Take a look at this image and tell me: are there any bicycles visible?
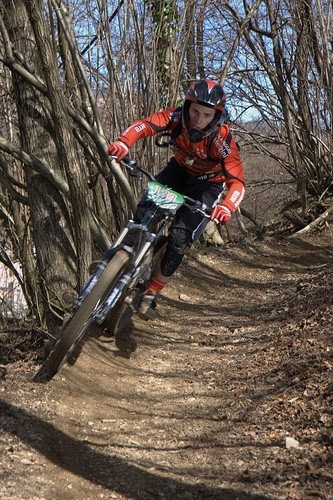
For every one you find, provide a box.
[46,152,220,377]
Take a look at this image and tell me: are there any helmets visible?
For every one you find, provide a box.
[182,80,225,143]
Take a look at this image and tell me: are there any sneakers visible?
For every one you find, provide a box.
[137,290,158,321]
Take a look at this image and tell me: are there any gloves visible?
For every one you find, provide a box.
[108,141,129,164]
[210,205,231,225]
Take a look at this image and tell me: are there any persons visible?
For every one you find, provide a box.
[88,78,246,316]
